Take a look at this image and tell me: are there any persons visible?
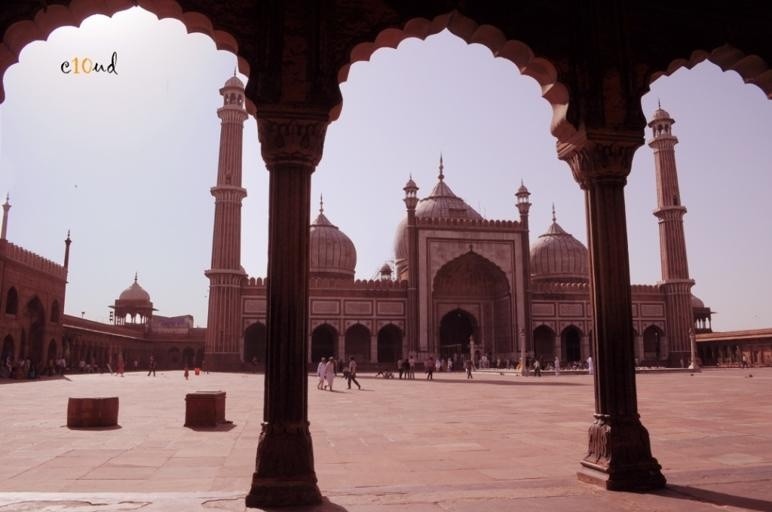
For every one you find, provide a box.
[6,354,67,380]
[114,353,126,377]
[679,353,685,368]
[687,357,695,369]
[347,355,361,389]
[397,352,562,379]
[586,354,593,376]
[93,361,99,371]
[741,352,749,368]
[146,355,157,376]
[316,356,326,390]
[80,356,86,373]
[184,364,190,380]
[633,355,640,366]
[324,357,336,391]
[333,358,337,375]
[135,357,138,371]
